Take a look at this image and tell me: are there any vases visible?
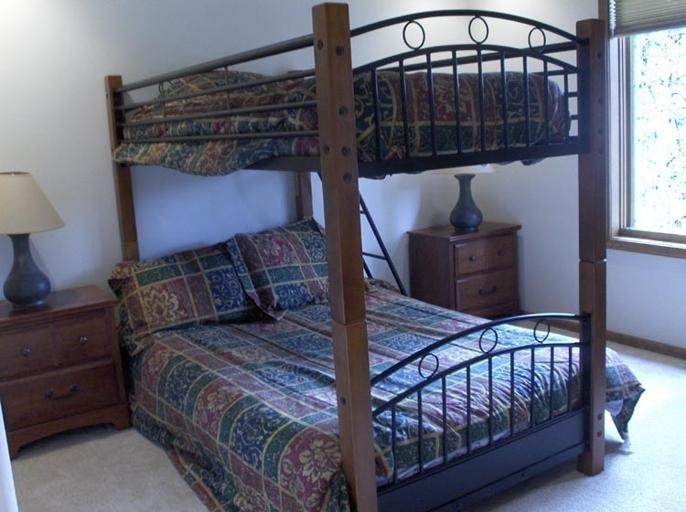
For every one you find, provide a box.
[450,173,482,233]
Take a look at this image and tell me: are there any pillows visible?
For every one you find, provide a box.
[155,70,304,106]
[225,219,376,321]
[106,240,264,357]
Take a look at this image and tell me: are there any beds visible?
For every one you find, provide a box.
[104,3,646,512]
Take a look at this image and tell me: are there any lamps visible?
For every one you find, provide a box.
[0,172,66,310]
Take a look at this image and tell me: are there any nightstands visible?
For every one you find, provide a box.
[0,284,129,460]
[405,221,522,320]
